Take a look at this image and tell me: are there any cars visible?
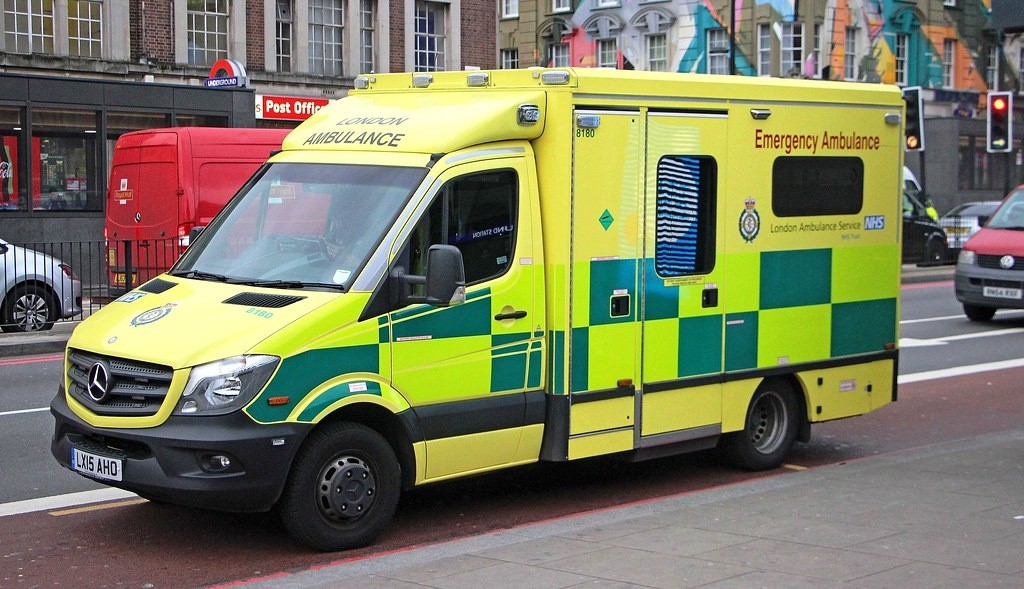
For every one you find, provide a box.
[938,201,1023,253]
[899,188,947,269]
[0,240,83,334]
[955,186,1024,324]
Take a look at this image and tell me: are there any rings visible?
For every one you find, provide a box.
[331,253,333,256]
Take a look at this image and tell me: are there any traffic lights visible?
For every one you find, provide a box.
[904,85,925,152]
[988,96,1010,153]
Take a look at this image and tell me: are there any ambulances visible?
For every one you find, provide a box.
[902,166,938,222]
[48,65,908,547]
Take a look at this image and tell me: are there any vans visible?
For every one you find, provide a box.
[105,125,332,303]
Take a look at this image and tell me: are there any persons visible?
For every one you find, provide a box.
[327,238,356,266]
[43,188,68,209]
